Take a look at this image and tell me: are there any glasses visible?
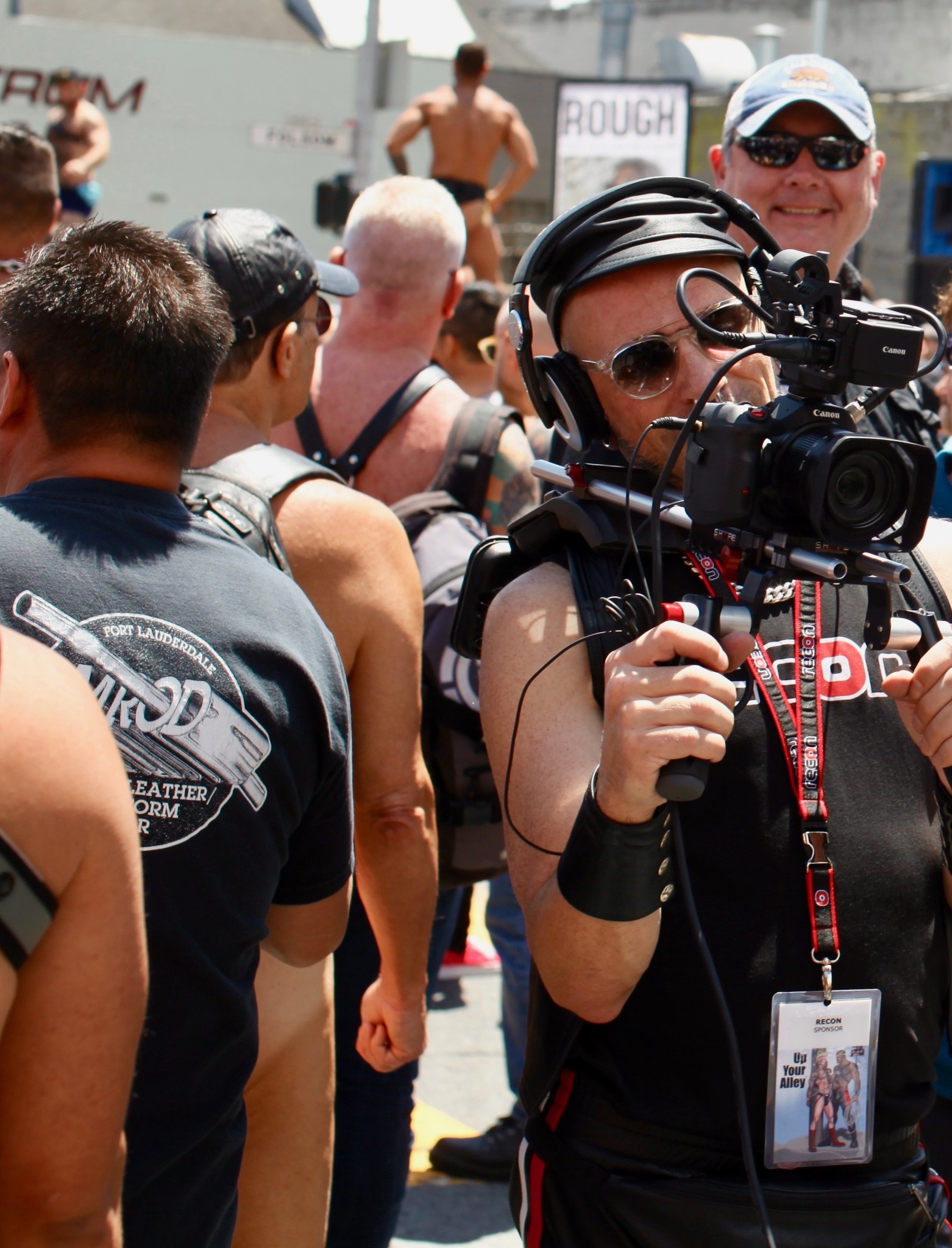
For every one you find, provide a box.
[729,130,869,171]
[289,295,332,336]
[579,286,761,400]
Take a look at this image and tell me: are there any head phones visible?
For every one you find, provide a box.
[511,177,799,451]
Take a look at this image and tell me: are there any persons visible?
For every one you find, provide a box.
[474,186,952,1248]
[384,42,539,283]
[707,51,951,520]
[0,125,555,1248]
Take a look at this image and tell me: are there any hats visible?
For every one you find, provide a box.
[167,208,359,344]
[722,54,876,150]
[530,193,747,352]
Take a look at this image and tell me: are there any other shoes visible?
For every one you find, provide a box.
[429,1098,533,1182]
[438,937,503,978]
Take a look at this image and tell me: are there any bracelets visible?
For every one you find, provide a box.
[558,767,678,922]
